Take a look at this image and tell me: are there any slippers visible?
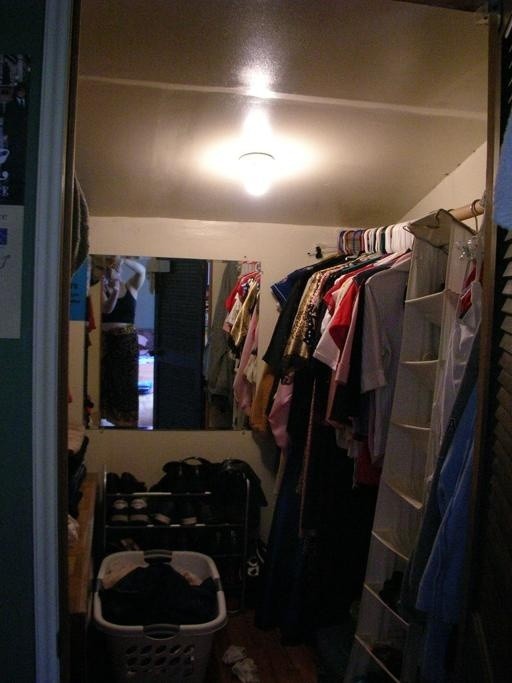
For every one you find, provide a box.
[226,596,247,614]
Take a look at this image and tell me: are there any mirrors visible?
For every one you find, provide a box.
[84,253,261,431]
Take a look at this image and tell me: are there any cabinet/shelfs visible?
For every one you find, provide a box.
[99,465,251,587]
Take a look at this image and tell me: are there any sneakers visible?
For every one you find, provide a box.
[105,461,243,525]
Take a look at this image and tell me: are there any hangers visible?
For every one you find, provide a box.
[236,262,260,292]
[271,221,415,309]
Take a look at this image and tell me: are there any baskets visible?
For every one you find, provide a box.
[94,550,228,683]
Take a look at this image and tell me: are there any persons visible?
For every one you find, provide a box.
[102,257,146,427]
